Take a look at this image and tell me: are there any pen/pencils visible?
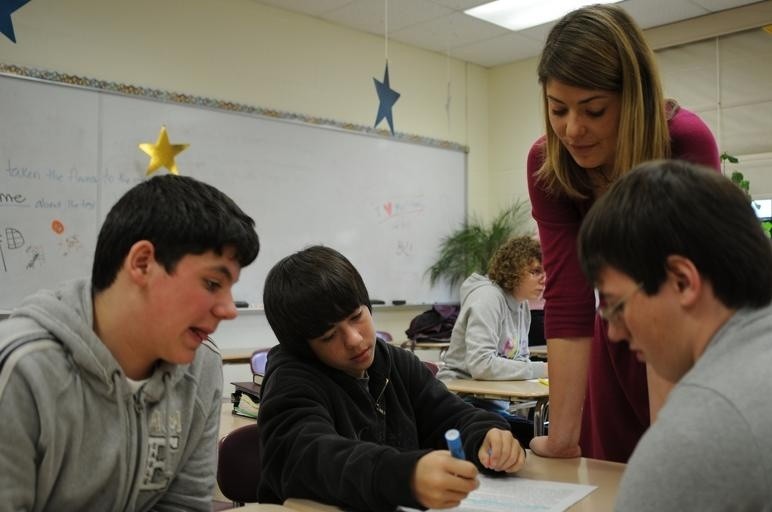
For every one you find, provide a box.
[444,428,466,461]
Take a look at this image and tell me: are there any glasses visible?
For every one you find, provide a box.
[529,269,549,279]
[598,282,643,322]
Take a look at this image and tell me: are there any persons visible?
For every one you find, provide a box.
[436,237,548,443]
[524,2,721,462]
[578,159,772,512]
[257,246,525,511]
[0,175,260,511]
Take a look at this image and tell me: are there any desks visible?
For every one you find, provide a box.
[220,450,632,512]
[394,343,546,432]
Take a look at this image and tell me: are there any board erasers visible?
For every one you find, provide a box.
[392,300,406,305]
[370,299,385,304]
[234,301,248,308]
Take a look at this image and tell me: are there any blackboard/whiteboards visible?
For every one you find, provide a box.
[0,64,468,321]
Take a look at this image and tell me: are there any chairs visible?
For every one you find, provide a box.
[218,342,287,504]
